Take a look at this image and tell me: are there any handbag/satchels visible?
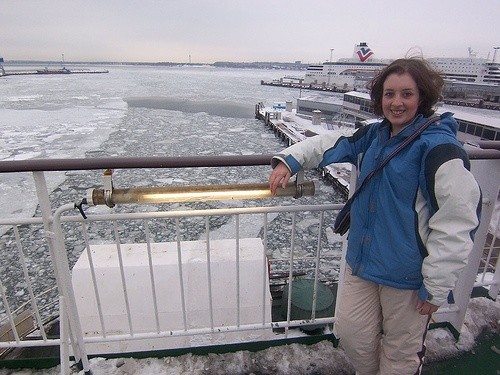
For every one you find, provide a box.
[333,191,351,237]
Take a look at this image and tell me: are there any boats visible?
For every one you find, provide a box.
[36,66,109,73]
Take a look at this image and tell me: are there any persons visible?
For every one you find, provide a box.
[269,55,481,375]
